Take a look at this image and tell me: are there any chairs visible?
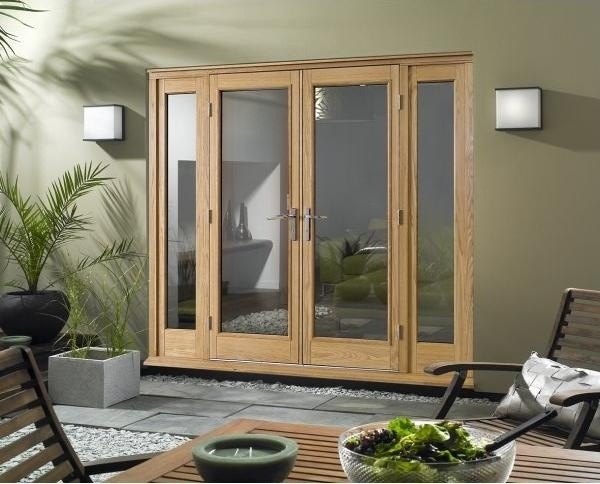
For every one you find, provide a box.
[0,345,166,483]
[424,287,600,450]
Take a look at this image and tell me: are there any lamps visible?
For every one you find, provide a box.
[82,104,125,142]
[495,85,544,132]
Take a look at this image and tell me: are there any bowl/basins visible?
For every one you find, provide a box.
[338,419,516,483]
[192,433,299,482]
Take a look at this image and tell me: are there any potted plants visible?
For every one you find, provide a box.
[1,158,138,345]
[48,264,142,411]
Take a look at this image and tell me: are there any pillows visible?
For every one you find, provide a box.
[491,349,600,441]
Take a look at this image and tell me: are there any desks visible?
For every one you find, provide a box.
[221,237,272,296]
